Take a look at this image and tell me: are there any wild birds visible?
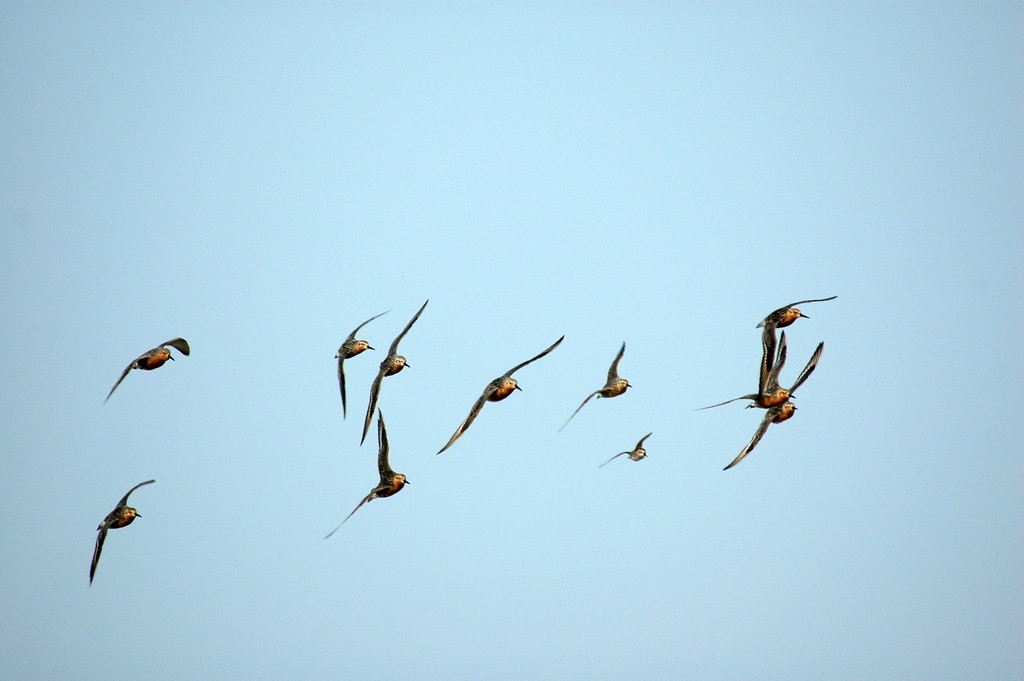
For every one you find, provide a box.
[87,480,156,589]
[101,337,191,405]
[599,431,654,467]
[334,299,443,446]
[436,333,567,459]
[693,292,838,471]
[556,341,634,432]
[323,408,410,541]
